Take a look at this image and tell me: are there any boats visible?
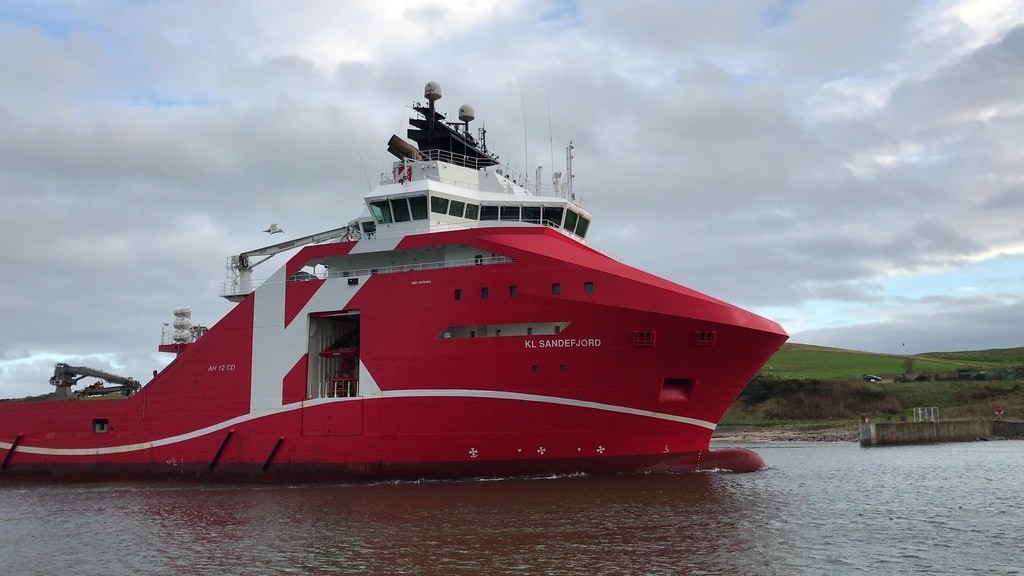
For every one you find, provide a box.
[0,82,789,486]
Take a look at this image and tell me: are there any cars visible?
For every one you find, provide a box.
[863,375,882,383]
